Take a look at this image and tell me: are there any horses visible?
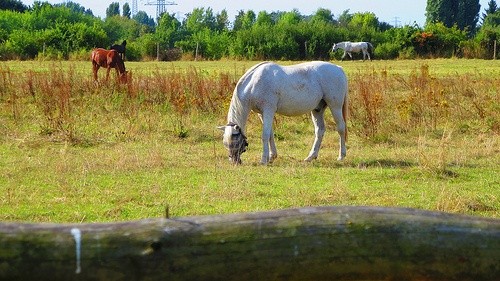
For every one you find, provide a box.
[90,40,128,81]
[219,61,349,164]
[331,41,374,62]
